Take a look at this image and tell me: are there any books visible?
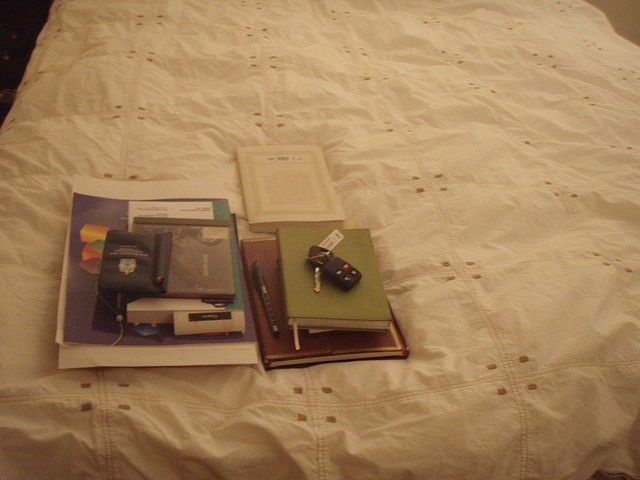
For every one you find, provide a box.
[237,142,346,236]
[242,237,411,371]
[277,224,392,336]
[124,199,246,326]
[126,216,235,301]
[54,173,261,371]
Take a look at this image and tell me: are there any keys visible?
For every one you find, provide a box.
[307,245,363,294]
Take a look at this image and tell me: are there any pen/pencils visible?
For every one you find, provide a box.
[250,260,281,338]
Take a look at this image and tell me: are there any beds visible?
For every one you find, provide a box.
[0,0,639,480]
